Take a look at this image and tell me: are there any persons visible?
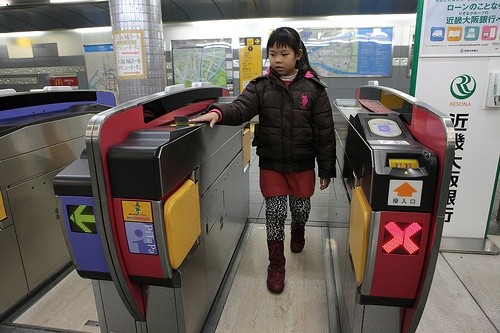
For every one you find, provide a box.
[186,26,336,295]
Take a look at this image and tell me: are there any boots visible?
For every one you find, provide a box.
[266,240,286,293]
[290,222,305,252]
